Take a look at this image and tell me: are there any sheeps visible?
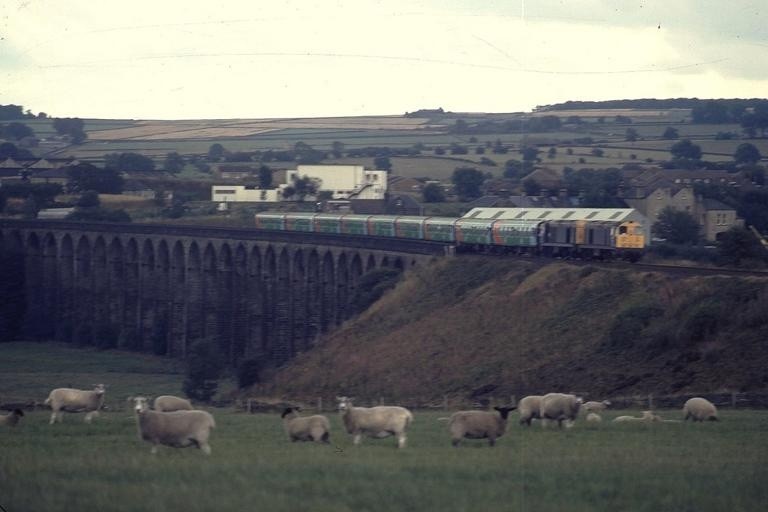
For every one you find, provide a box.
[280,405,332,443]
[44,382,110,424]
[680,394,719,422]
[0,406,27,428]
[333,395,415,450]
[446,405,518,447]
[518,390,662,428]
[126,394,217,454]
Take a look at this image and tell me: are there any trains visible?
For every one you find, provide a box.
[253,206,650,263]
